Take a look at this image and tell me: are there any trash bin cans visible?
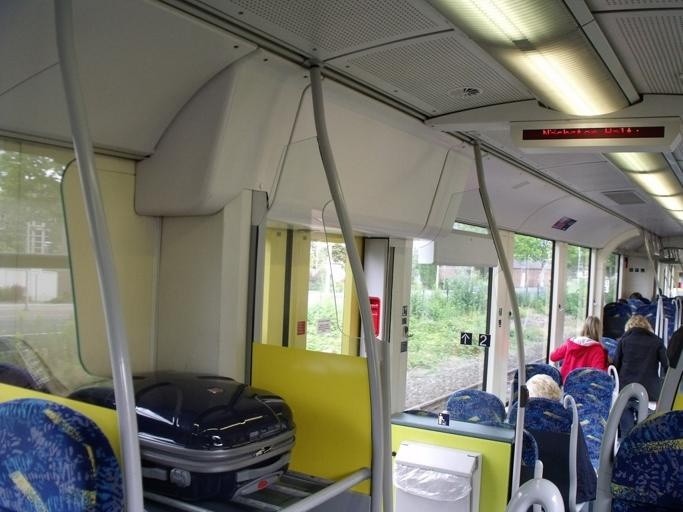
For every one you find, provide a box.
[394,441,483,512]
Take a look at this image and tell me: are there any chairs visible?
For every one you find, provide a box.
[592,383,683,512]
[602,299,683,368]
[506,394,598,512]
[564,362,619,467]
[511,364,562,397]
[480,418,538,487]
[450,387,506,427]
[0,399,126,512]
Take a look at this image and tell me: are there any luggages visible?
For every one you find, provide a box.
[67,372,297,503]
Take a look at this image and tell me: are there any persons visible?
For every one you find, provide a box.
[550,315,608,388]
[524,373,561,401]
[614,314,669,441]
